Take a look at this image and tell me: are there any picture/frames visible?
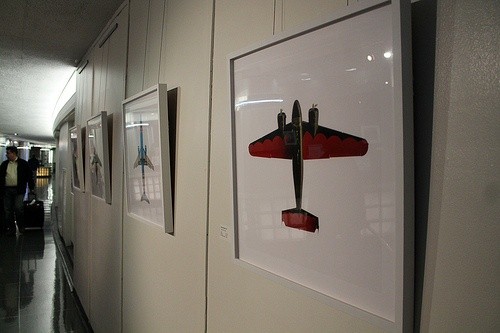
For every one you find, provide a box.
[224,0,416,333]
[85,110,111,205]
[118,82,175,234]
[67,123,85,195]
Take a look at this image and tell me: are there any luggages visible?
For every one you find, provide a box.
[20,196,44,230]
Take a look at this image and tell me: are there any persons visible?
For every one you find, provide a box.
[0,147,34,233]
[27,152,40,187]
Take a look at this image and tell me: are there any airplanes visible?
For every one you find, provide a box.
[132,115,156,205]
[91,146,103,186]
[246,101,368,232]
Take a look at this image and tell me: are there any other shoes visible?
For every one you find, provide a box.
[8,223,16,233]
[16,222,23,233]
